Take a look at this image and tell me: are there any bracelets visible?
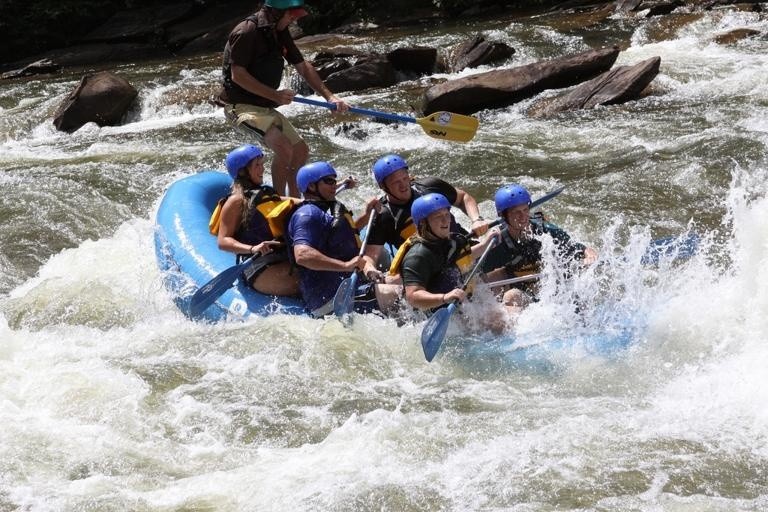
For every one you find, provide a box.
[248,245,254,254]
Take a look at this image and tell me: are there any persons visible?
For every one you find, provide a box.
[481,183,600,309]
[288,159,380,318]
[215,0,349,198]
[359,155,487,308]
[213,146,359,298]
[401,193,476,311]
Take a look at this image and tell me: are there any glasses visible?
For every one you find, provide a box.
[323,178,336,184]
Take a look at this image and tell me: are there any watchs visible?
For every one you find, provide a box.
[471,216,484,223]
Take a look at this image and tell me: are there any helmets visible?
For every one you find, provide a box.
[374,155,408,189]
[225,144,263,179]
[296,162,337,193]
[495,184,532,216]
[264,0,309,17]
[411,193,451,229]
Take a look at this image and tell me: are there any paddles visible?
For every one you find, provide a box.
[188,176,351,316]
[332,208,376,327]
[420,235,498,364]
[293,97,478,143]
[464,186,566,239]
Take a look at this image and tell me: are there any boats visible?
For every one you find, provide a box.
[153,171,648,385]
